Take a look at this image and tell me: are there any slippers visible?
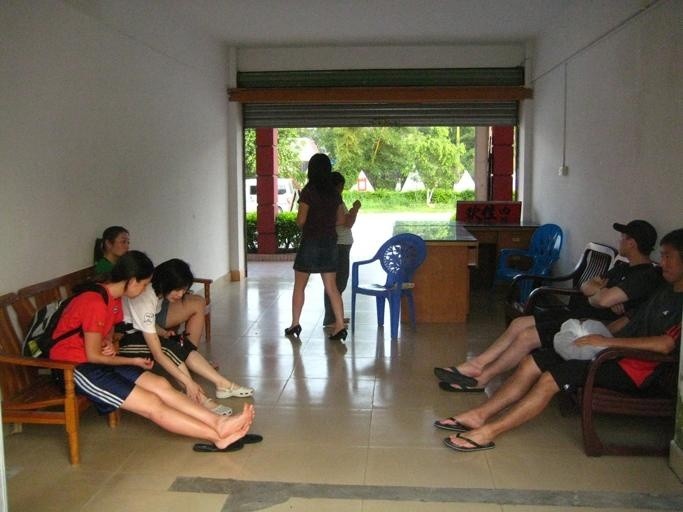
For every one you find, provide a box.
[434,367,478,387]
[442,433,496,452]
[433,417,471,432]
[237,434,263,443]
[193,442,243,452]
[438,382,485,392]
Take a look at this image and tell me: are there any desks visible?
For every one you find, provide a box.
[394,225,479,323]
[395,219,541,254]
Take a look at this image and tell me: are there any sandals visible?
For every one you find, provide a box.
[201,398,233,417]
[216,382,255,399]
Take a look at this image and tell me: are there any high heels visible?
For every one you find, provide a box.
[285,325,301,338]
[328,328,347,340]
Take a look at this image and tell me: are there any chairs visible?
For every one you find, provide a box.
[351,232,426,339]
[1,265,213,465]
[492,223,563,302]
[505,242,679,457]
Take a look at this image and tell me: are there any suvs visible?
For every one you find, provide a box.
[244,177,300,215]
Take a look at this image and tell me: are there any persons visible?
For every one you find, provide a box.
[432,228,683,452]
[434,219,661,393]
[93,225,206,349]
[47,250,256,451]
[323,171,361,330]
[114,257,255,416]
[284,154,346,342]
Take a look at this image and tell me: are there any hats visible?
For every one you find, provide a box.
[613,220,657,251]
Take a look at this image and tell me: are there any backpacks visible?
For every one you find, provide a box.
[22,285,109,359]
